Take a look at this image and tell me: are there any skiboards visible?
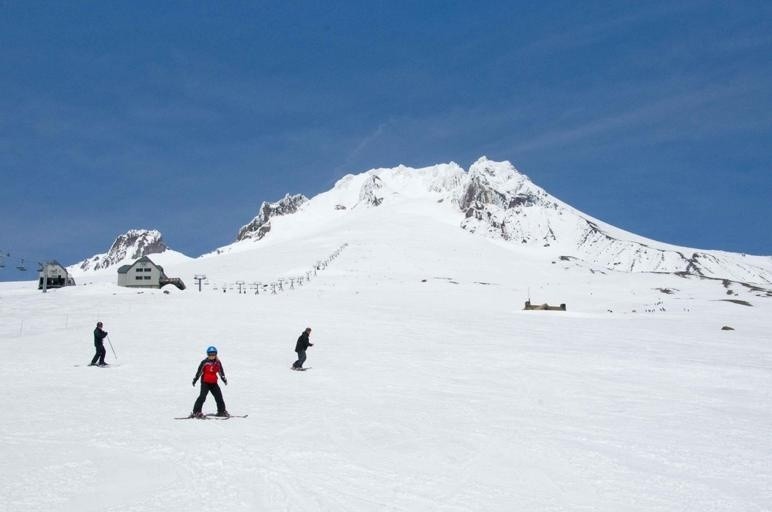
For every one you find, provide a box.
[172,414,248,421]
[289,367,312,371]
[73,363,122,369]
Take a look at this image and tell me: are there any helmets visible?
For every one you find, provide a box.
[206,346,218,353]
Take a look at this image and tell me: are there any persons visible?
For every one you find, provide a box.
[292,327,315,372]
[91,322,109,367]
[193,345,231,420]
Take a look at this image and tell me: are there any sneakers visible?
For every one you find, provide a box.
[193,410,230,419]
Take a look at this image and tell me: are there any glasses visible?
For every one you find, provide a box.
[208,353,216,356]
